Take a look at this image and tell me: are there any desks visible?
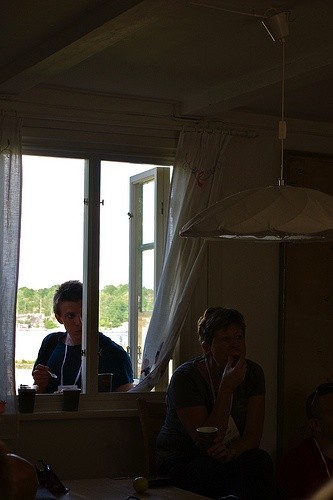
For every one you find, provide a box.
[61,475,214,500]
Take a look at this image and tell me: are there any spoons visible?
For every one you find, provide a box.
[38,364,57,378]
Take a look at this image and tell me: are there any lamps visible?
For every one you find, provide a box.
[179,10,333,244]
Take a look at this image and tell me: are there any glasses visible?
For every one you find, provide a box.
[309,383,333,407]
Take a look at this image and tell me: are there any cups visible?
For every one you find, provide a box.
[196,427,218,455]
[63,389,81,412]
[98,372,113,392]
[58,386,73,389]
[18,389,36,413]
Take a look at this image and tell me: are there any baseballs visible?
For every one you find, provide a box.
[132,477,149,493]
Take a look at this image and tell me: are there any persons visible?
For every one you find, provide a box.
[31,282,134,393]
[269,380,333,500]
[155,306,290,500]
